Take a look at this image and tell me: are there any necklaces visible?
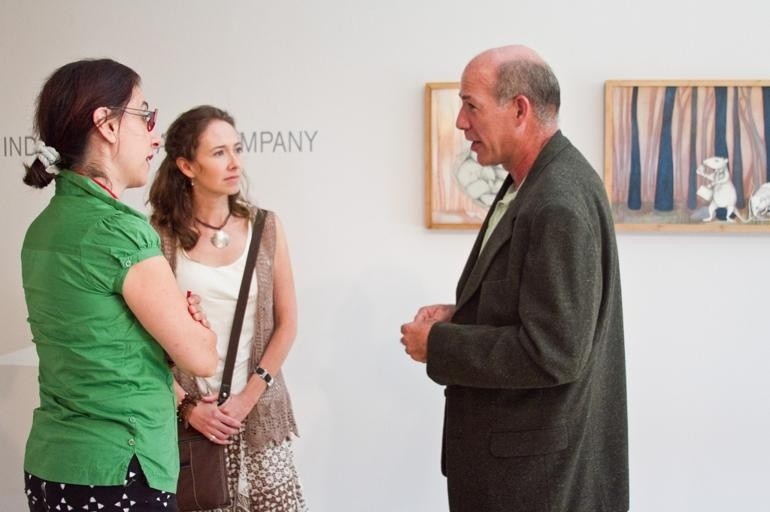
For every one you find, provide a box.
[191,207,234,250]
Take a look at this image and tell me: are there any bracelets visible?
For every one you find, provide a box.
[176,389,203,424]
[250,362,276,389]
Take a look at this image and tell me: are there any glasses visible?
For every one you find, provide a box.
[107,106,157,132]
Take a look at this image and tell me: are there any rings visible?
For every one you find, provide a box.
[209,435,215,442]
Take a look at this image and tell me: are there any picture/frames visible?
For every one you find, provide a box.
[600,79,770,235]
[423,83,504,229]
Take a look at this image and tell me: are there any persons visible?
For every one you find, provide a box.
[19,57,219,512]
[399,43,630,512]
[148,104,310,512]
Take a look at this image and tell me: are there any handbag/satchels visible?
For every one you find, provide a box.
[177,422,231,510]
